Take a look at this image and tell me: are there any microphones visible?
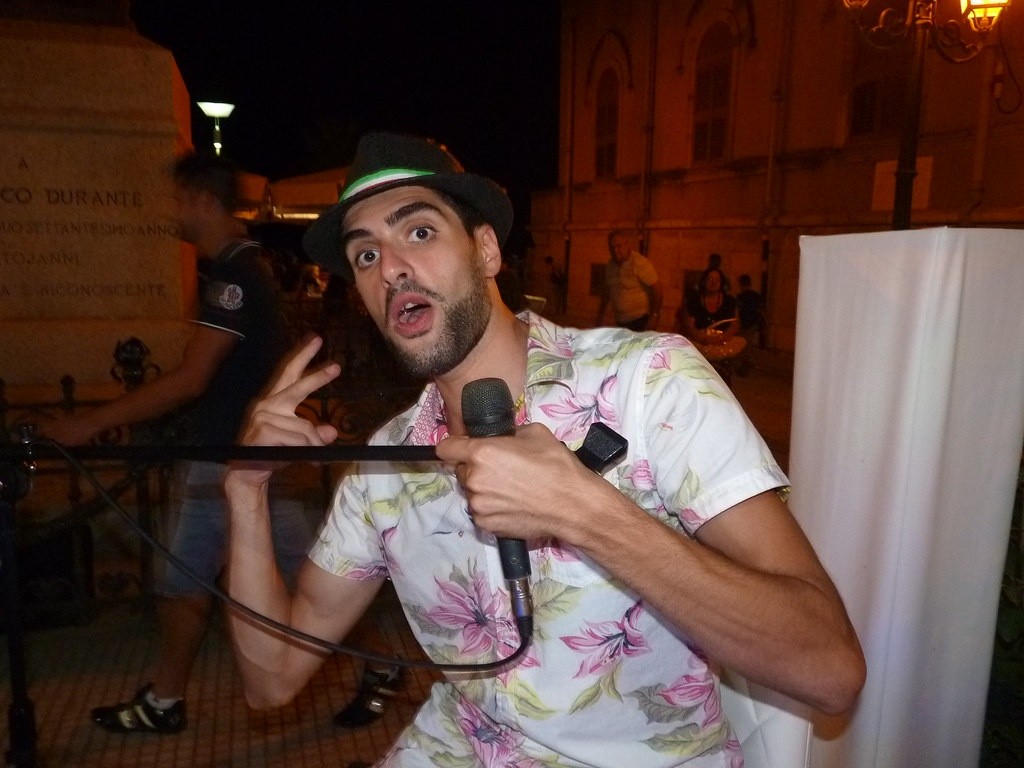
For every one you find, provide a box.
[461,377,533,641]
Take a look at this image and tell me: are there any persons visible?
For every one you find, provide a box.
[50,150,411,735]
[226,129,868,768]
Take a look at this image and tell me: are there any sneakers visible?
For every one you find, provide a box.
[332,661,409,729]
[90,681,188,735]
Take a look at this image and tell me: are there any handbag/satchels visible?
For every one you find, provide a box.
[690,318,747,360]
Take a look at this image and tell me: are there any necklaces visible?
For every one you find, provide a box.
[704,293,720,323]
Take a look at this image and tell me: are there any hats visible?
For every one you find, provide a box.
[305,133,513,281]
